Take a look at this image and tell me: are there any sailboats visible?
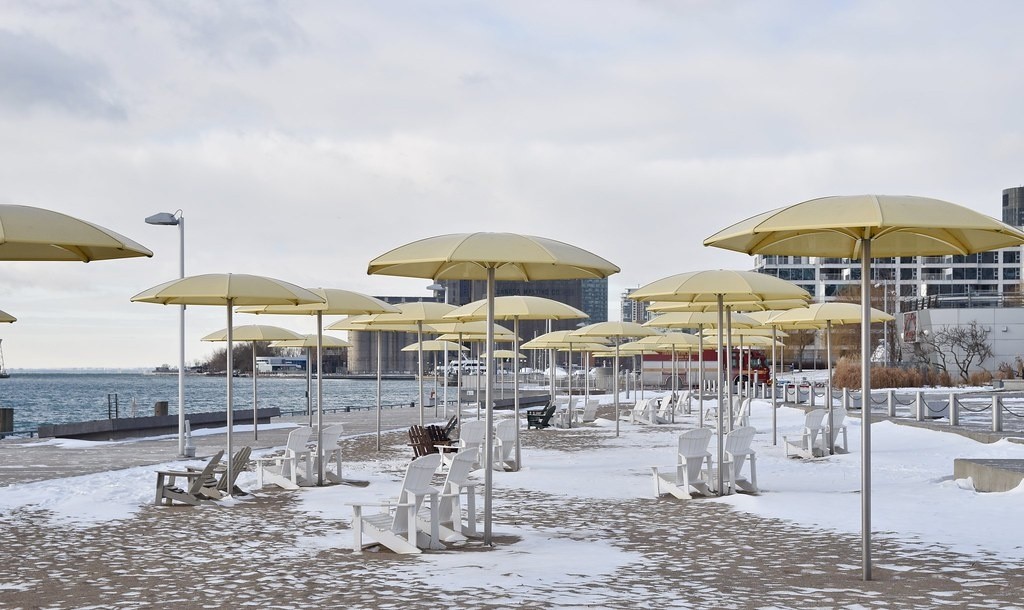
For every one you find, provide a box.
[0,339,10,378]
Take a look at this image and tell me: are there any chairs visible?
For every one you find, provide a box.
[152,390,850,556]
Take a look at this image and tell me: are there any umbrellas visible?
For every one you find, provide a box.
[367,232,620,549]
[0,310,17,323]
[0,204,153,263]
[627,269,897,496]
[267,334,354,428]
[323,302,523,451]
[234,288,403,488]
[129,272,326,497]
[520,322,790,436]
[441,296,589,472]
[199,325,307,440]
[703,195,1024,582]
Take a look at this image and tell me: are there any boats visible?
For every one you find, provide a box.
[436,361,486,372]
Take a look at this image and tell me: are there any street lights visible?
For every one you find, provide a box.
[576,323,589,403]
[145,209,188,458]
[874,280,887,367]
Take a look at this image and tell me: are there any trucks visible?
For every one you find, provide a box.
[643,346,772,391]
